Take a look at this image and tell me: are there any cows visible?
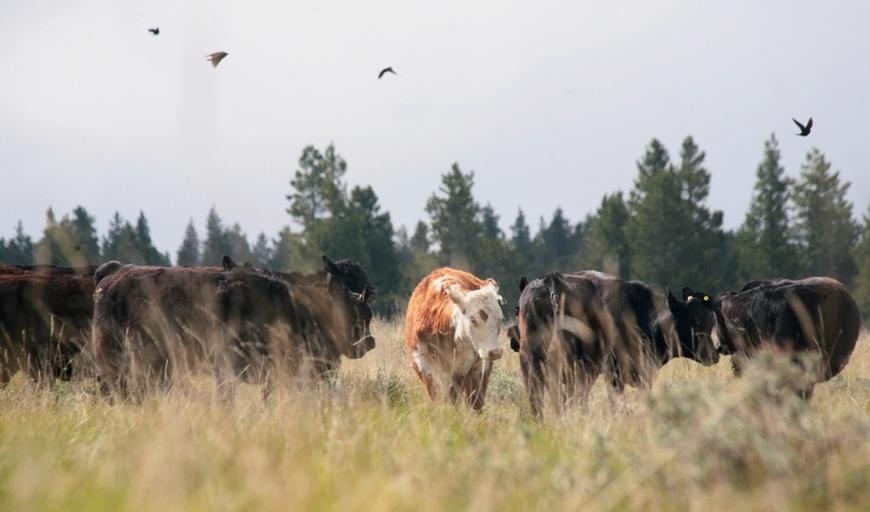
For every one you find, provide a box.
[510,270,863,422]
[0,251,378,401]
[401,262,506,414]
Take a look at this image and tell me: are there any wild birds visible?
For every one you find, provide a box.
[207,51,229,68]
[148,27,161,34]
[377,66,396,79]
[793,118,813,137]
